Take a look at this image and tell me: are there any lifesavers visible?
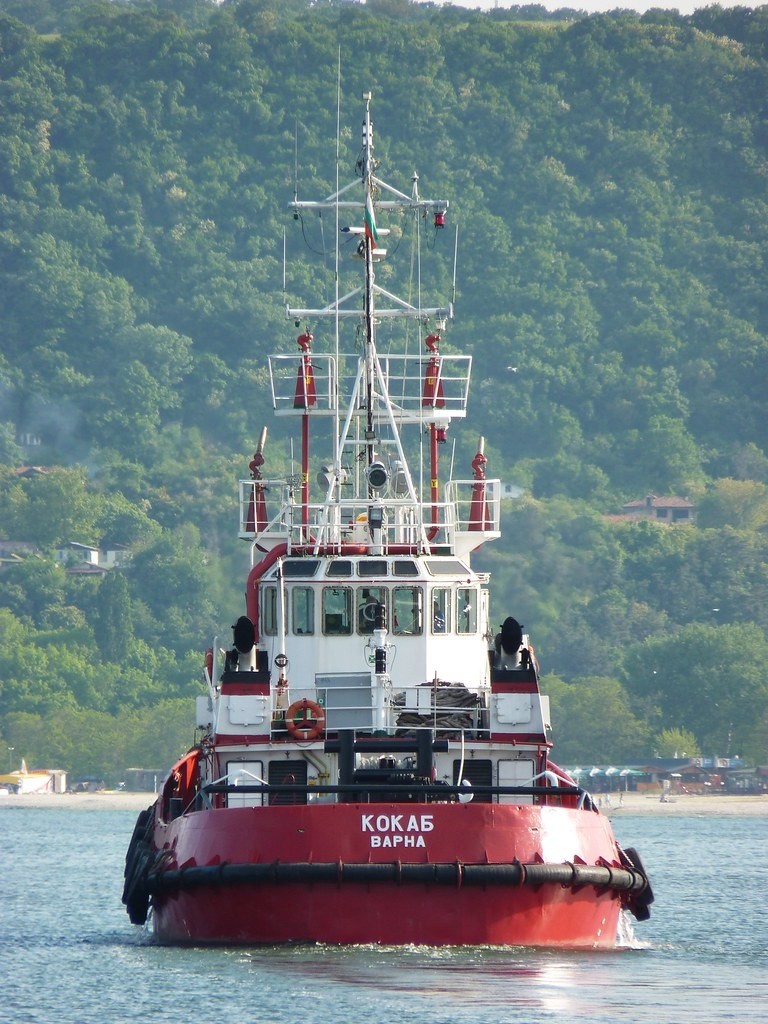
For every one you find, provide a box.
[205,647,213,680]
[120,807,151,927]
[620,845,655,905]
[636,905,651,921]
[284,698,325,742]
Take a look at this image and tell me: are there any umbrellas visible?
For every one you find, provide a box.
[562,765,645,794]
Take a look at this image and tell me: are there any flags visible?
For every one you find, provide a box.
[364,194,379,249]
[21,757,27,775]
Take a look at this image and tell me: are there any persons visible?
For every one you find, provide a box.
[605,792,609,808]
[599,796,602,807]
[434,601,445,633]
[619,793,624,805]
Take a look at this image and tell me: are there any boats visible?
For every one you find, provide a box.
[122,50,663,959]
